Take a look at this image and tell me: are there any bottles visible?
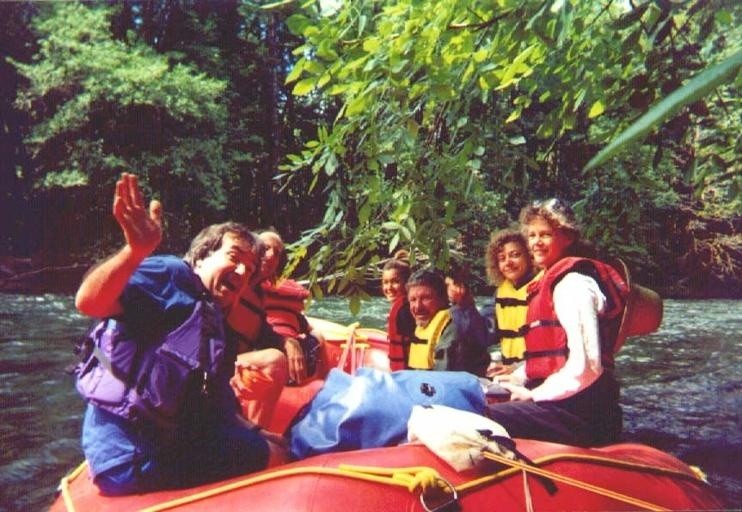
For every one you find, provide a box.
[489,352,503,367]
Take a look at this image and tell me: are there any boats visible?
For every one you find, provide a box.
[48,315,727,512]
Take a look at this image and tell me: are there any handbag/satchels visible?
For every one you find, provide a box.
[405,397,561,496]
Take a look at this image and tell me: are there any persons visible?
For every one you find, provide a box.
[259,275,322,384]
[381,259,415,371]
[483,229,542,382]
[73,167,269,497]
[217,221,283,355]
[478,198,625,447]
[402,269,492,377]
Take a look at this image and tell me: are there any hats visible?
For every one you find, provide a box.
[603,252,663,354]
